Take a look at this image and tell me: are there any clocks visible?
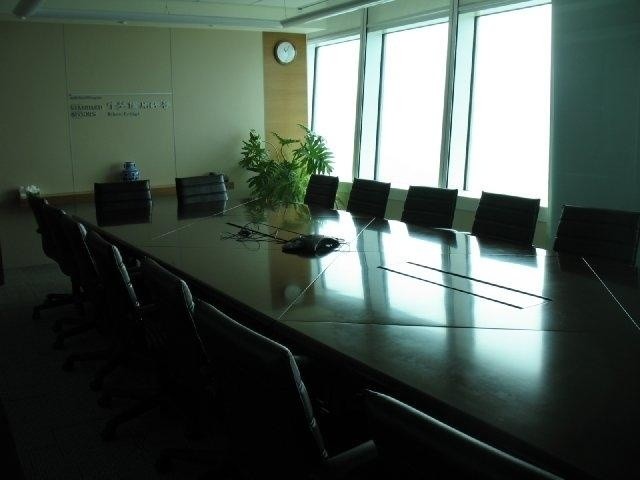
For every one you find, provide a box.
[275,39,297,66]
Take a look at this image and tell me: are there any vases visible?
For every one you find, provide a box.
[121,162,140,181]
